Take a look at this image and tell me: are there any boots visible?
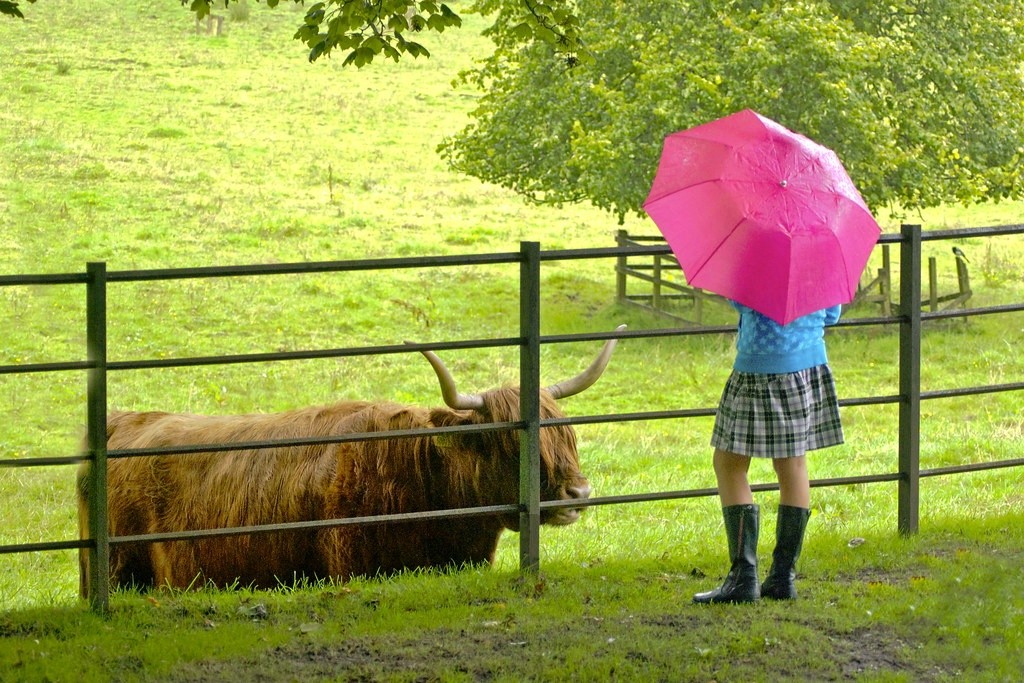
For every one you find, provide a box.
[759,504,812,601]
[691,504,761,606]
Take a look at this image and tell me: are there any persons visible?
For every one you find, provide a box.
[696,298,846,604]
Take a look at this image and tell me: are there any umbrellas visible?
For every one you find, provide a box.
[641,106,883,327]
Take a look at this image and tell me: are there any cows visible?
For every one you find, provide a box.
[76,322,628,597]
